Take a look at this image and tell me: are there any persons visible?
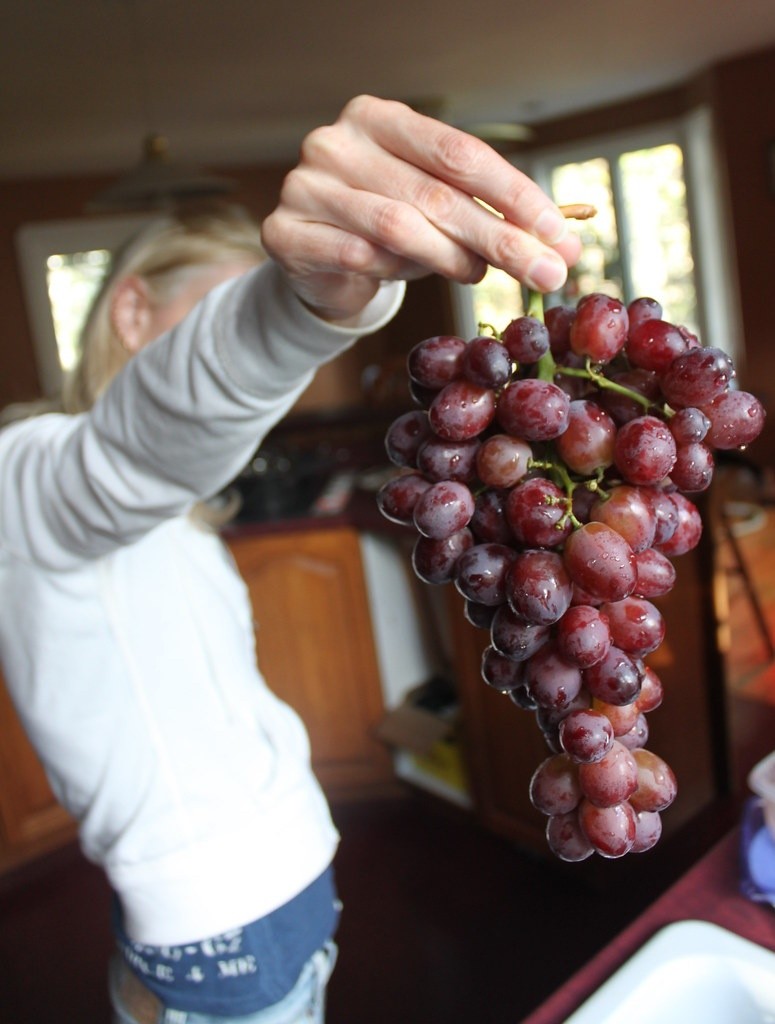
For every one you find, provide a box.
[0,94,584,1024]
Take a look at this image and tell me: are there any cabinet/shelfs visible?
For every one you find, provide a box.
[446,471,716,875]
[0,512,397,890]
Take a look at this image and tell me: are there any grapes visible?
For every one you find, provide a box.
[376,292,768,864]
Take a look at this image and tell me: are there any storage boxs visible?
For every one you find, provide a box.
[369,673,481,815]
